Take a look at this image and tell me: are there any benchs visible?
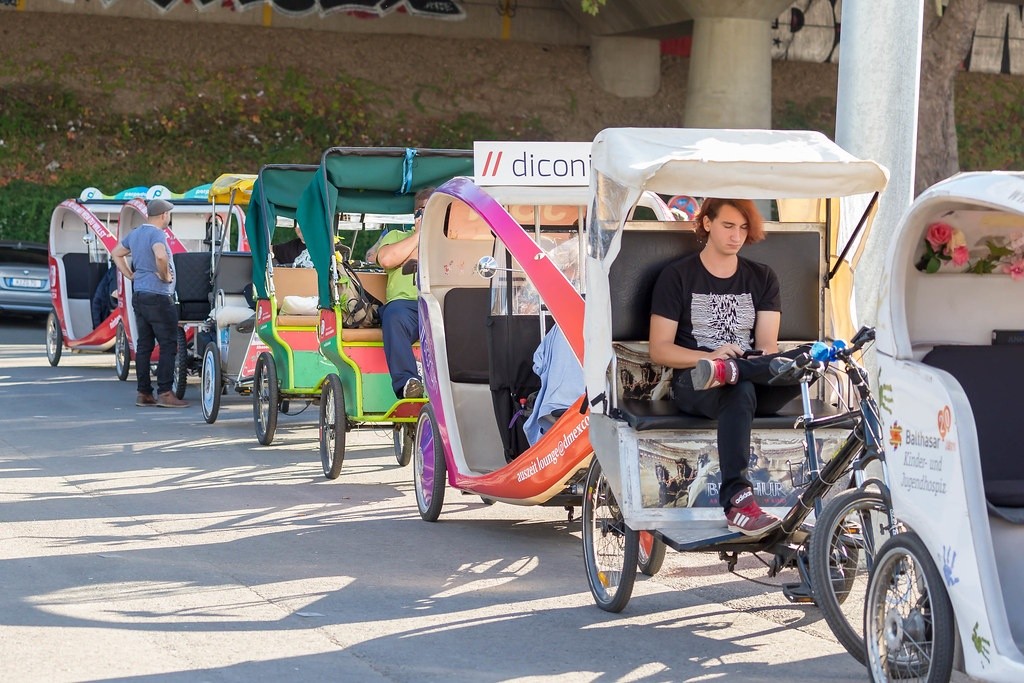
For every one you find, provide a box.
[443,287,538,385]
[62,252,108,299]
[208,253,257,328]
[593,229,857,431]
[338,272,420,342]
[267,267,320,327]
[173,252,251,320]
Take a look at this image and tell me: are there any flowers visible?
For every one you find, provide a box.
[962,231,1024,279]
[915,223,970,275]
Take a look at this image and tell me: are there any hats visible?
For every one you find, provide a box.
[147,199,173,216]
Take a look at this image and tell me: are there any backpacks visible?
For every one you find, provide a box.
[337,261,383,329]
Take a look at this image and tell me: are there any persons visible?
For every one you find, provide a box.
[365,229,388,263]
[376,188,435,400]
[112,199,190,408]
[236,220,307,334]
[650,197,829,536]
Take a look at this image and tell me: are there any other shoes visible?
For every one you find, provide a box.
[403,377,424,398]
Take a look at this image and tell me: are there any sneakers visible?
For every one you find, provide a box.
[136,391,158,406]
[728,487,781,536]
[156,391,191,407]
[690,359,739,390]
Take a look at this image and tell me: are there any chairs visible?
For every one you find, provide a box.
[991,329,1024,344]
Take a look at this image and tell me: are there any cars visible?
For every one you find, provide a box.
[0,239,54,321]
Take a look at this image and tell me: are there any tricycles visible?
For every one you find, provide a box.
[580,127,947,678]
[412,172,666,579]
[854,169,1024,683]
[299,147,472,481]
[47,163,341,446]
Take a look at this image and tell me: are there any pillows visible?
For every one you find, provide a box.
[279,295,320,316]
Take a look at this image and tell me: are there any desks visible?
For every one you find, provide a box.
[920,344,1024,524]
